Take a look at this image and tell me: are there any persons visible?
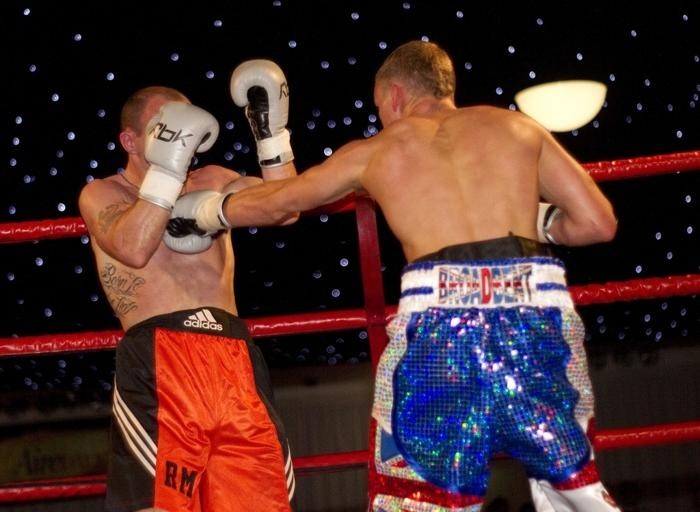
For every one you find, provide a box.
[161,40,619,512]
[77,56,301,512]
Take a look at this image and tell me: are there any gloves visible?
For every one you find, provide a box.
[162,189,234,254]
[230,60,296,169]
[138,101,220,212]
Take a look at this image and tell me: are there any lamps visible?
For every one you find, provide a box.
[514,73,607,133]
